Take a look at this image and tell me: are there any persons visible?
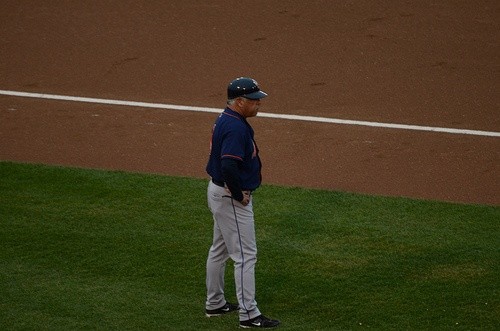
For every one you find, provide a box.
[204,77,280,331]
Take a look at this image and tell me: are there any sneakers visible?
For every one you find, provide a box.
[240,315,280,329]
[204,300,240,317]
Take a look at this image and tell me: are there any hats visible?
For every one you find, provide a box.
[227,77,268,99]
